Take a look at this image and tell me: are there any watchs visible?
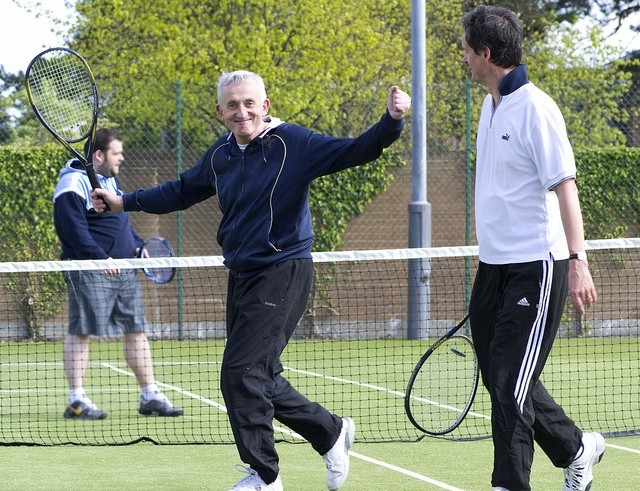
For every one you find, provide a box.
[569,252,587,261]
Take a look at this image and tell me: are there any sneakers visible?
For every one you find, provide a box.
[63,398,107,420]
[321,416,356,491]
[228,464,284,490]
[139,394,184,416]
[562,431,606,490]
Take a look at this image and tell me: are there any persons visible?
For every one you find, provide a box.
[460,4,607,491]
[89,69,412,491]
[52,127,184,420]
